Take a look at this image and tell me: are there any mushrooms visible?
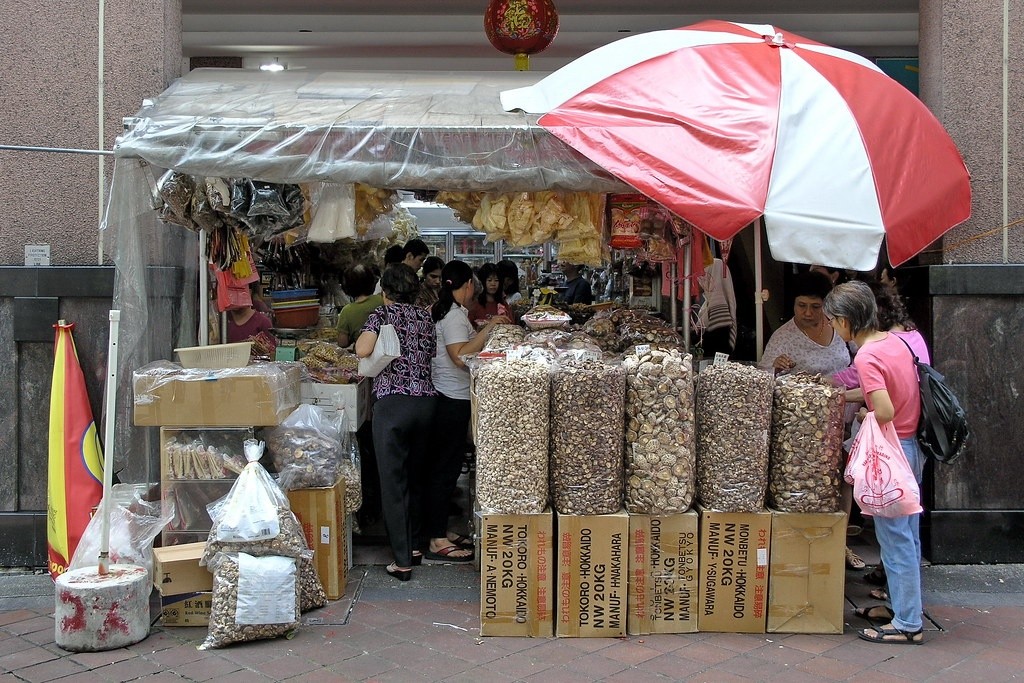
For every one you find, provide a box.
[773,371,847,513]
[624,343,695,516]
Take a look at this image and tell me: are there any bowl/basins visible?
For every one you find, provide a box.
[270,290,321,327]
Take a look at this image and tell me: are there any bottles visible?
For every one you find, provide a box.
[463,237,482,254]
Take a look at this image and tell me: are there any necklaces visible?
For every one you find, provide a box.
[796,317,824,340]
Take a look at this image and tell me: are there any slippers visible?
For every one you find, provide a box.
[453,535,473,547]
[425,546,474,562]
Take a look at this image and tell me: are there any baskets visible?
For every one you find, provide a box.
[593,302,614,312]
[174,342,255,368]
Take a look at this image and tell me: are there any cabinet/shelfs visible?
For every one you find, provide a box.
[160,426,255,547]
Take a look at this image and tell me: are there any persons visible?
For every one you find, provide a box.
[353,263,437,581]
[336,239,525,473]
[227,283,270,342]
[560,261,592,305]
[759,272,868,568]
[805,265,933,326]
[425,259,511,563]
[823,273,931,644]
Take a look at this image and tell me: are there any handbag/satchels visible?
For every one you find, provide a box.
[843,412,923,517]
[917,361,969,464]
[358,311,401,377]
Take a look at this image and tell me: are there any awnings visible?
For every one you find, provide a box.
[119,69,645,194]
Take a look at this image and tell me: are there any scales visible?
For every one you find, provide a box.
[268,328,311,361]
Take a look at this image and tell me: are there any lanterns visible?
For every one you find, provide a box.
[485,0,559,71]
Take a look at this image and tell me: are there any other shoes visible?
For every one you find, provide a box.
[846,523,864,535]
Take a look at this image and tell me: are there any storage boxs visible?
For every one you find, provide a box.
[283,478,350,602]
[556,515,628,636]
[631,511,697,635]
[479,510,552,641]
[130,366,301,428]
[769,509,848,633]
[301,377,370,433]
[156,591,214,627]
[149,541,218,594]
[701,509,772,635]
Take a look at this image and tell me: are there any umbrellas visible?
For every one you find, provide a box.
[44,320,104,588]
[500,21,975,366]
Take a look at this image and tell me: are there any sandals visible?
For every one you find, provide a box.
[845,549,866,570]
[869,585,889,600]
[386,561,411,581]
[856,605,893,619]
[413,556,422,566]
[863,565,886,581]
[858,626,924,644]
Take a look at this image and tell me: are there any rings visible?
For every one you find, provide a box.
[780,357,782,360]
